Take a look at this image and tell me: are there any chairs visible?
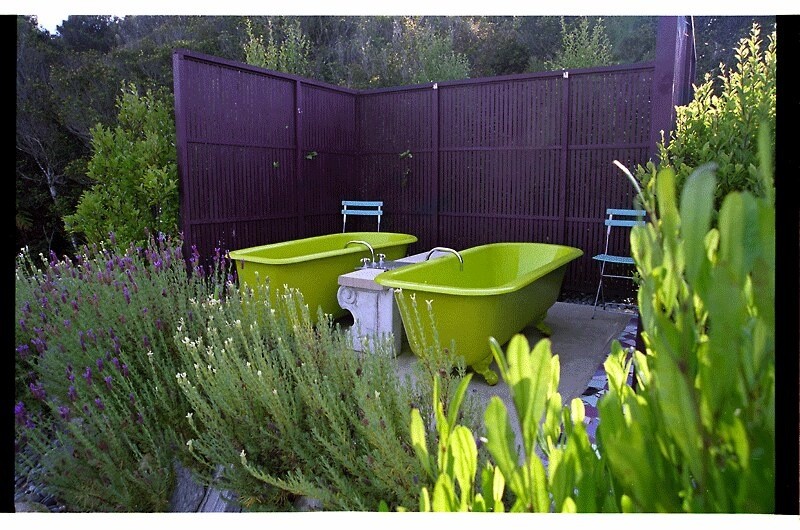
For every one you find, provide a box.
[592,209,646,320]
[341,201,383,232]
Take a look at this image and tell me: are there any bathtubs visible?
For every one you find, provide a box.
[225,232,418,330]
[374,242,584,386]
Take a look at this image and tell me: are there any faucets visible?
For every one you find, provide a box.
[425,247,463,264]
[343,240,375,262]
